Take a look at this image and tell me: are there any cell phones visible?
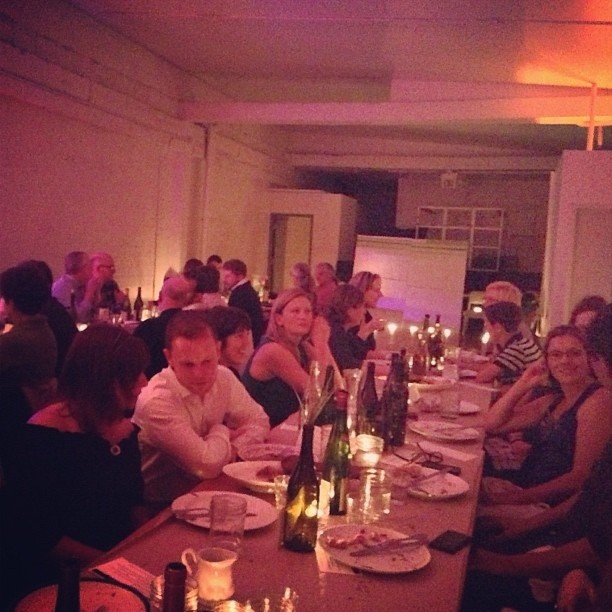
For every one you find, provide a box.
[421,459,462,476]
[429,529,474,556]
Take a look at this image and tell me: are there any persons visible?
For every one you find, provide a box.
[205,255,223,268]
[347,271,388,362]
[314,262,339,314]
[521,290,538,325]
[51,251,102,322]
[482,281,532,358]
[182,258,204,278]
[0,266,58,385]
[184,265,226,309]
[289,262,316,293]
[133,277,190,381]
[131,311,271,518]
[85,253,132,313]
[461,301,543,385]
[205,305,253,393]
[570,295,606,336]
[15,321,149,563]
[319,284,367,372]
[474,325,611,526]
[19,259,80,369]
[223,259,265,349]
[241,287,341,429]
[473,302,611,610]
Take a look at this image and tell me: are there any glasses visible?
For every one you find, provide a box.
[97,265,116,271]
[390,440,443,464]
[548,346,585,361]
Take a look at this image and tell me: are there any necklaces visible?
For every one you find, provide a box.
[104,436,123,455]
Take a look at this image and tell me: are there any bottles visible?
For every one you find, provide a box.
[310,365,337,425]
[358,349,409,446]
[284,425,319,552]
[162,563,186,612]
[322,390,350,514]
[413,314,443,374]
[299,360,321,428]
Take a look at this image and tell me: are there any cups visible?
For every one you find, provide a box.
[210,494,247,556]
[181,548,239,601]
[351,469,392,519]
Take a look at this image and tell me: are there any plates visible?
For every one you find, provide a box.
[459,401,481,414]
[407,467,470,502]
[410,420,480,444]
[171,491,278,530]
[317,523,431,575]
[223,461,291,492]
[237,444,318,461]
